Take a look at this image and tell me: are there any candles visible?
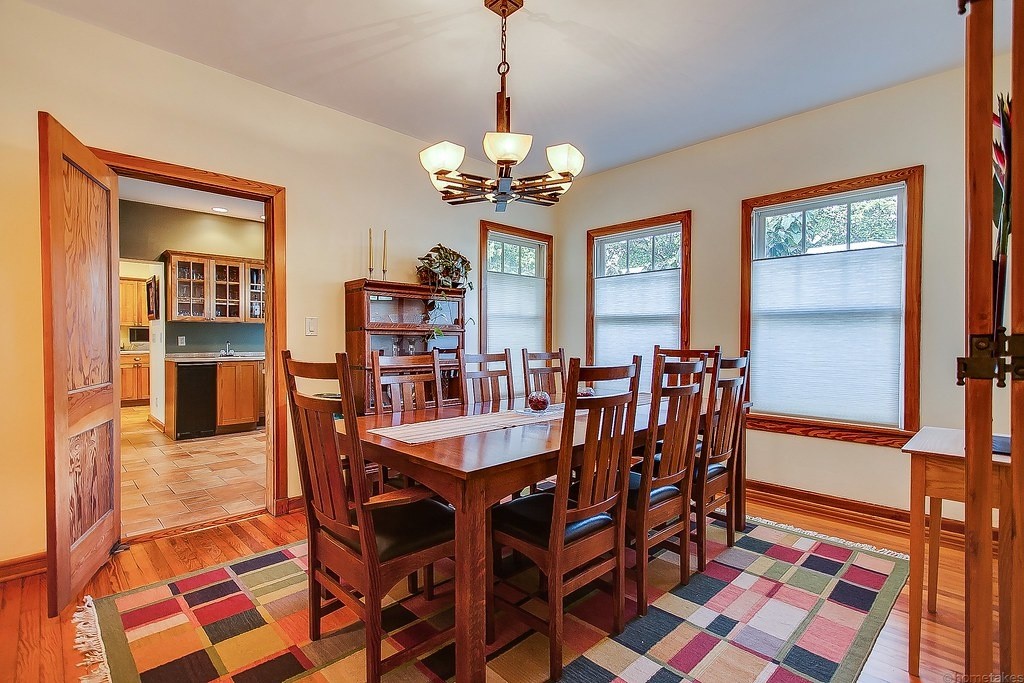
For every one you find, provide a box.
[369,227,373,268]
[383,230,387,270]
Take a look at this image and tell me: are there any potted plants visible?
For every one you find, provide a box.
[414,243,475,343]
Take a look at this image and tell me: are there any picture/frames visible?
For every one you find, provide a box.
[146,275,157,320]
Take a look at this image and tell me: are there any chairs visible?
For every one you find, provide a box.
[282,344,751,683]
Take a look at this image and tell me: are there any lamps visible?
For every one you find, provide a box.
[418,0,586,212]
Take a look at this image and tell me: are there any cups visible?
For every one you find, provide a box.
[392,338,399,356]
[407,338,416,355]
[379,350,384,356]
[178,260,239,316]
[251,271,258,290]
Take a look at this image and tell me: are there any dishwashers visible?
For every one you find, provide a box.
[177,362,218,440]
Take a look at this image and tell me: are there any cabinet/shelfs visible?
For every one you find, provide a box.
[243,258,266,323]
[120,277,149,326]
[120,354,150,401]
[344,279,466,418]
[217,361,259,426]
[161,250,245,323]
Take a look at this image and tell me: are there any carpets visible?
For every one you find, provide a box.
[71,506,911,683]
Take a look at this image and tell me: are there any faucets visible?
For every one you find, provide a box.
[227,340,231,355]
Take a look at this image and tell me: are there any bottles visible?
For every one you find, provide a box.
[252,295,260,317]
[262,304,265,318]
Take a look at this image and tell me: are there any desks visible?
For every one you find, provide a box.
[902,425,1010,683]
[322,389,754,683]
